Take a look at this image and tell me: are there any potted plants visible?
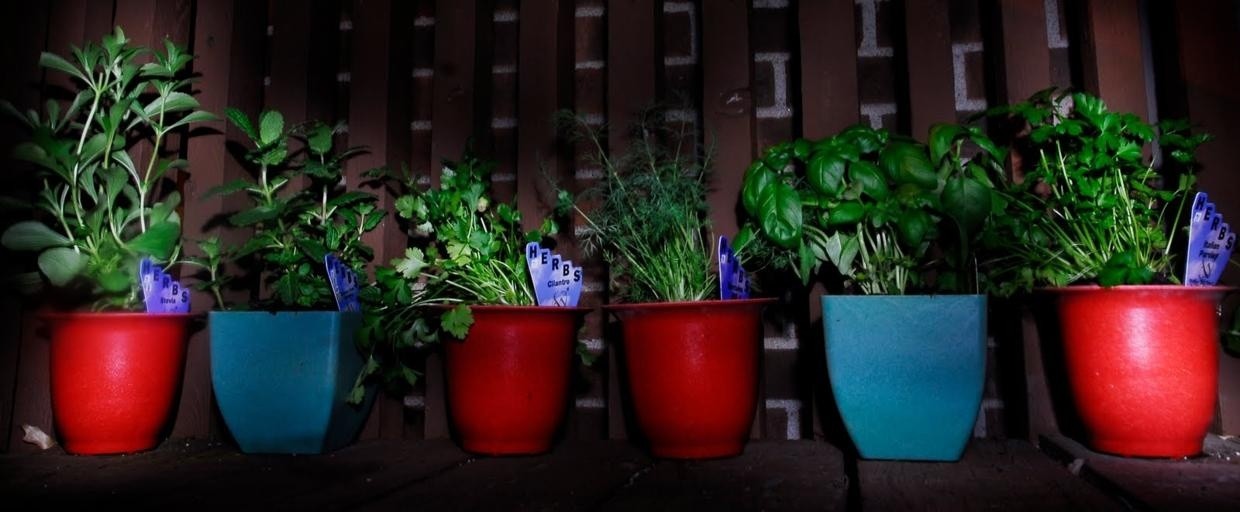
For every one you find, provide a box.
[963,87,1240,459]
[1,25,220,454]
[534,97,782,460]
[344,134,595,455]
[736,125,1005,462]
[176,107,388,455]
